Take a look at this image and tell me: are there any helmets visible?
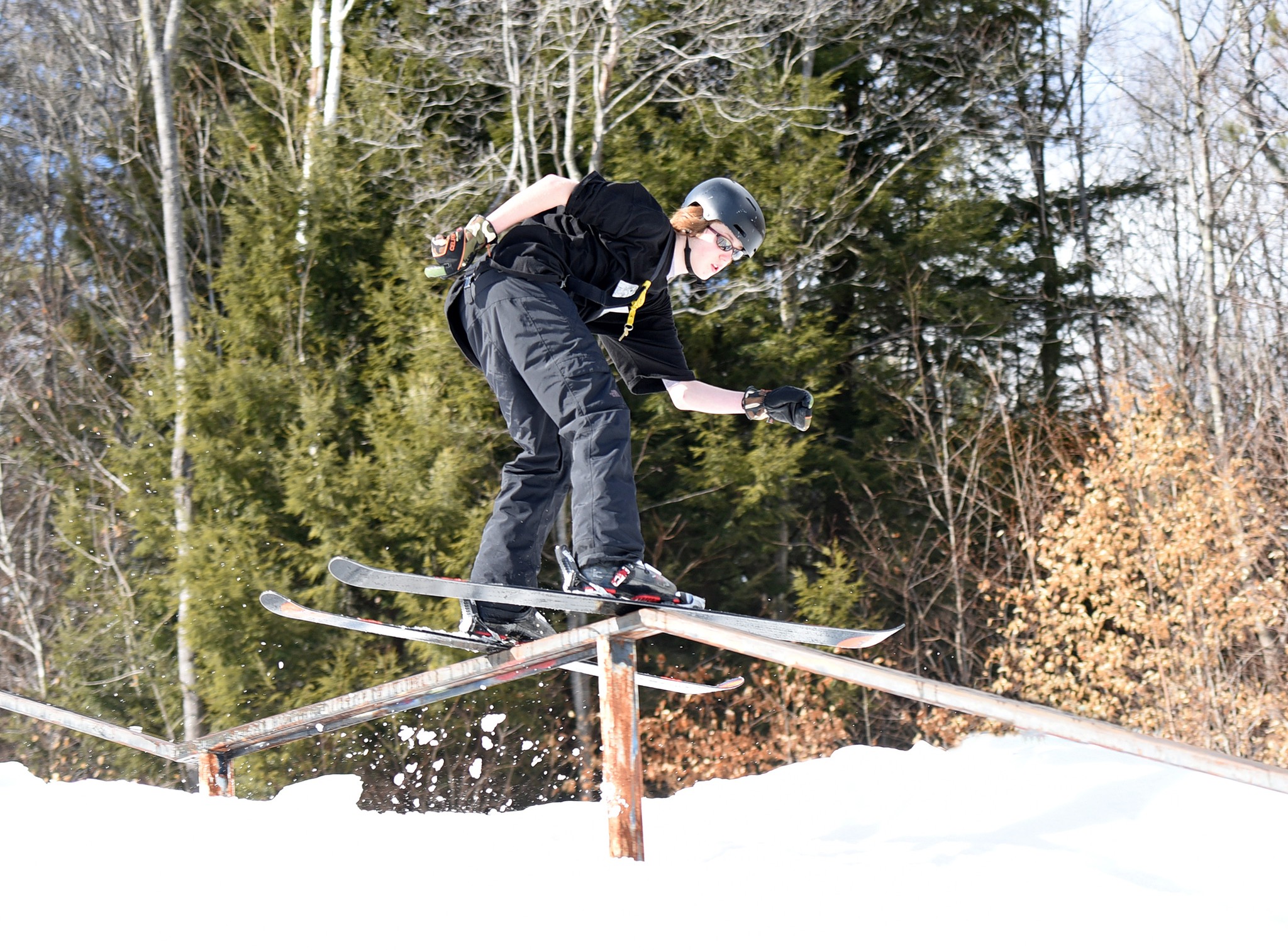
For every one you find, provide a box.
[680,177,766,260]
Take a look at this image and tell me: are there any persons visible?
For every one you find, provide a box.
[424,172,815,647]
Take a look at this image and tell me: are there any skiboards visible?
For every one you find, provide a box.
[258,554,906,695]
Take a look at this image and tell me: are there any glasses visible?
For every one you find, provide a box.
[705,225,744,262]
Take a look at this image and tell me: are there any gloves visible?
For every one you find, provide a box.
[424,213,499,280]
[742,385,814,432]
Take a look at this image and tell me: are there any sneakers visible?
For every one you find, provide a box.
[458,598,558,647]
[555,544,706,610]
